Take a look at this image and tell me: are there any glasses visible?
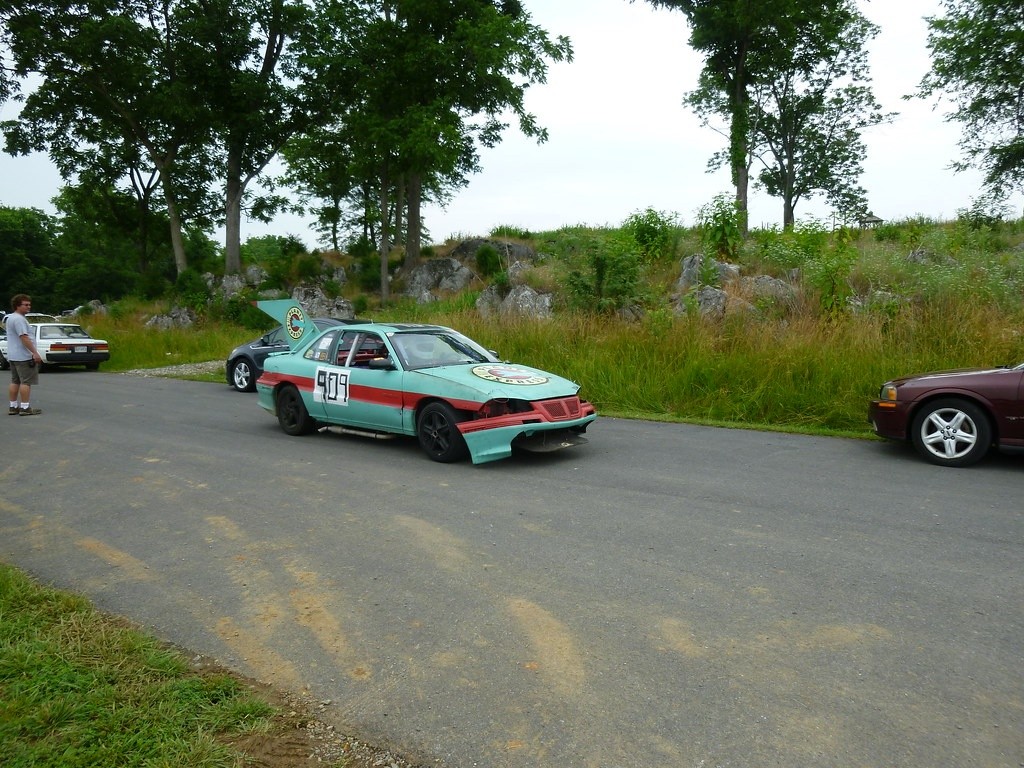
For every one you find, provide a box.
[21,304,31,306]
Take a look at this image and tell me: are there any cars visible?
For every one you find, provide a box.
[0,310,111,373]
[226,316,385,394]
[865,361,1024,469]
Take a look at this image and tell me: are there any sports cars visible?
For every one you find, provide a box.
[250,297,597,464]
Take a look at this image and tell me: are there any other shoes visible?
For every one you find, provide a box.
[18,405,41,415]
[9,406,19,414]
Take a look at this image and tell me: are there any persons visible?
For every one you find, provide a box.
[6,294,41,416]
[408,335,435,360]
